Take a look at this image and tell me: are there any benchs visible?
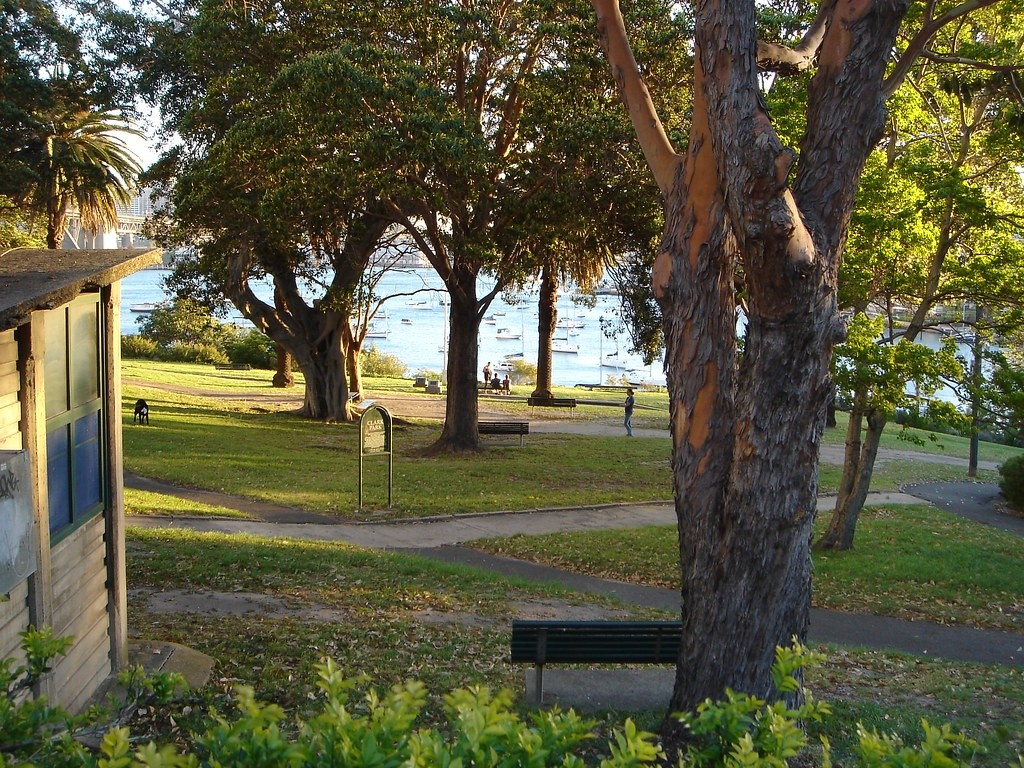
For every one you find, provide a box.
[478,383,510,395]
[508,618,686,712]
[215,363,251,375]
[478,422,530,448]
[528,397,577,417]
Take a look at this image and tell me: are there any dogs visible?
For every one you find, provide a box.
[133,399,150,424]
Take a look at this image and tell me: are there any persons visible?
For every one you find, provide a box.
[483,362,511,394]
[624,388,634,436]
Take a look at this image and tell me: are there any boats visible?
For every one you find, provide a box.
[482,299,531,325]
[405,295,453,310]
[602,323,657,386]
[493,340,526,372]
[352,290,394,339]
[401,318,413,325]
[130,301,162,313]
[495,327,523,339]
[550,294,586,353]
